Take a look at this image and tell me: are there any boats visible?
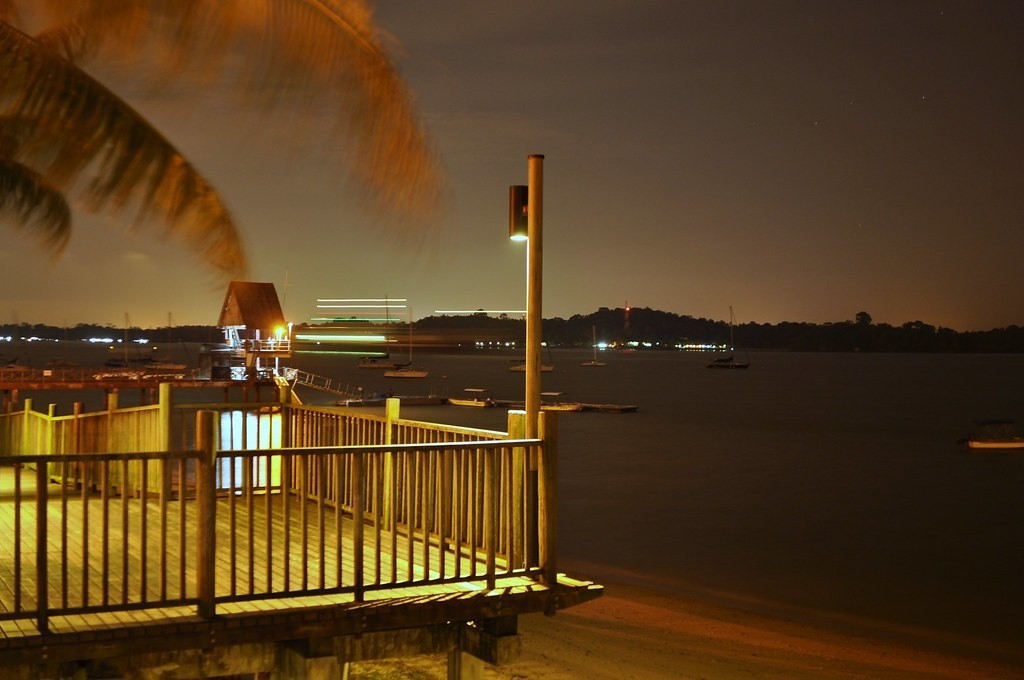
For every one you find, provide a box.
[538,391,583,412]
[447,388,496,408]
[362,389,443,407]
[954,417,1024,451]
[579,403,639,413]
[494,399,526,409]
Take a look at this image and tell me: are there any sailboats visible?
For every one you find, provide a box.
[383,304,429,378]
[508,338,554,372]
[706,305,751,369]
[356,295,410,370]
[91,312,189,381]
[581,325,606,366]
